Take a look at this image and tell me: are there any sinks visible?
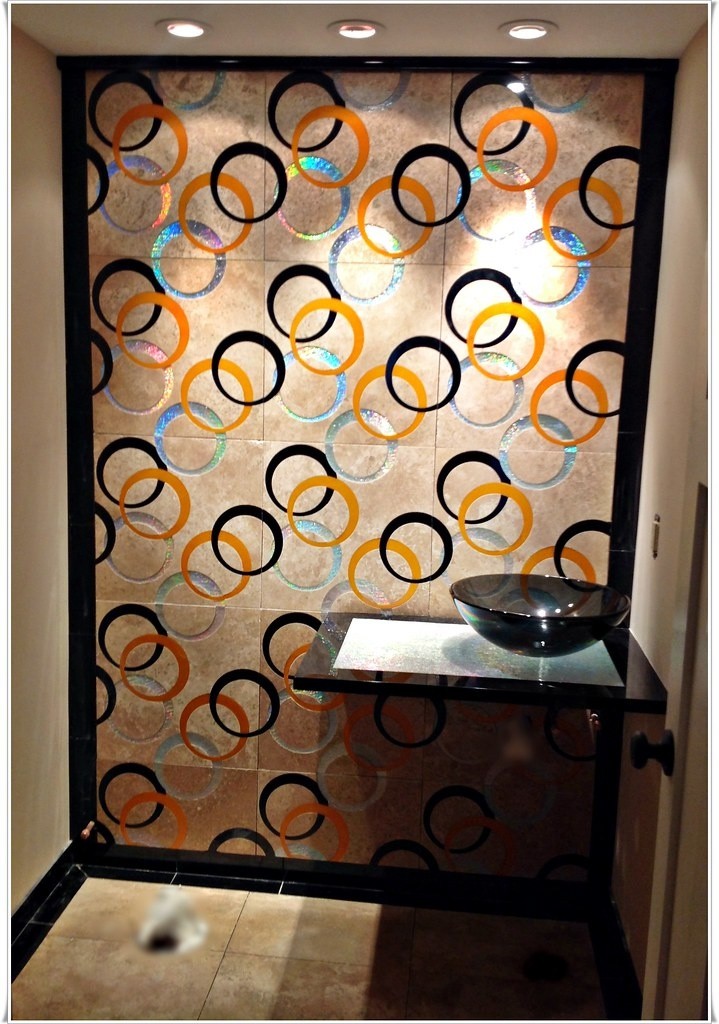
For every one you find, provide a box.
[450,572,631,658]
